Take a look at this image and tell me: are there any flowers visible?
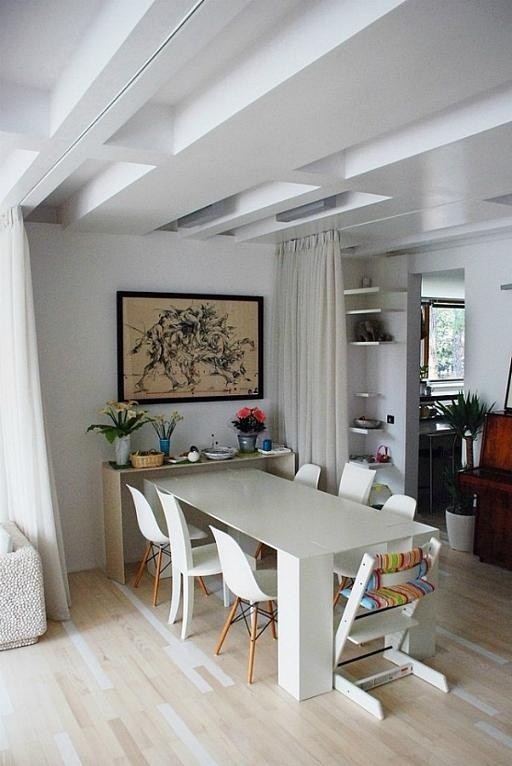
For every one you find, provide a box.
[85,400,157,444]
[230,406,267,433]
[148,410,184,439]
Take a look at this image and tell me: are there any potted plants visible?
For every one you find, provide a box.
[431,387,496,552]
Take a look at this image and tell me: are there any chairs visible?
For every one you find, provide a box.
[208,524,278,685]
[154,484,230,641]
[338,462,377,506]
[126,483,209,608]
[333,495,418,610]
[333,537,449,721]
[293,463,321,490]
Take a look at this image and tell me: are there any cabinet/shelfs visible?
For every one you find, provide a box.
[454,410,512,572]
[419,435,457,515]
[100,448,296,585]
[343,277,407,507]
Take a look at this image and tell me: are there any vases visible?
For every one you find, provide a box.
[160,439,170,456]
[237,434,257,452]
[114,435,130,465]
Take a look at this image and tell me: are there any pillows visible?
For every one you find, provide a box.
[1,528,13,555]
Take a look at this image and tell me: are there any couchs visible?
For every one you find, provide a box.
[1,519,46,652]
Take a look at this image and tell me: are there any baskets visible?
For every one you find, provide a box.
[376,443,389,462]
[129,448,165,468]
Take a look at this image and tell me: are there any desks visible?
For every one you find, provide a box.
[143,466,439,703]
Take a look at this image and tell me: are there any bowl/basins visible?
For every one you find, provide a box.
[203,447,238,459]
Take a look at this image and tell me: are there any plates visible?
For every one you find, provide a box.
[164,456,188,464]
[258,447,291,454]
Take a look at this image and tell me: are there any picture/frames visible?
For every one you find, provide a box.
[116,290,264,405]
